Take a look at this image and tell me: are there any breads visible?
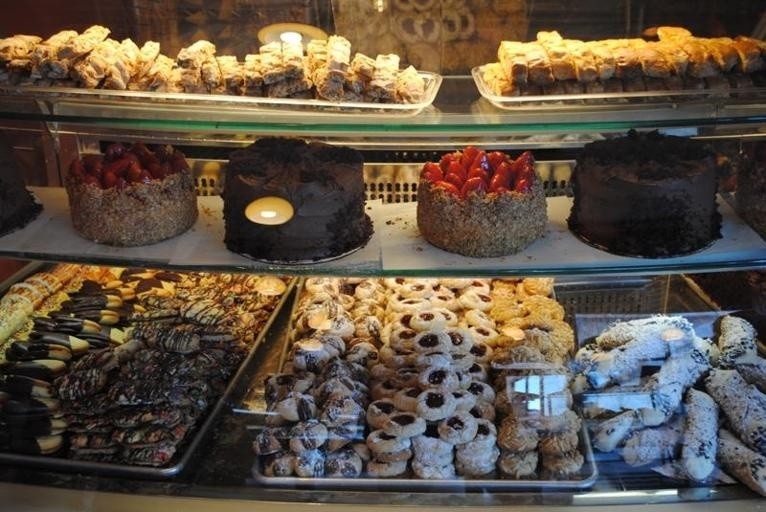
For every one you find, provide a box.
[572,313,765,498]
[483,24,765,107]
[0,263,294,467]
[0,22,425,107]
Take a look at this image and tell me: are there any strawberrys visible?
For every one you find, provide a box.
[66,141,190,189]
[421,147,538,197]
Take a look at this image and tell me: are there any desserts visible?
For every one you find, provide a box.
[253,276,583,479]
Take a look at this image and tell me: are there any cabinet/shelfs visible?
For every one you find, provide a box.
[0,75,766,512]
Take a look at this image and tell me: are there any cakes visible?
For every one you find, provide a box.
[221,137,375,265]
[62,141,198,247]
[0,124,43,234]
[415,145,551,257]
[565,125,722,260]
